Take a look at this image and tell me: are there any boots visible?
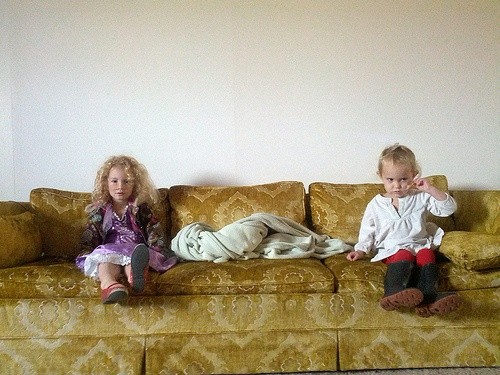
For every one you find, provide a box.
[414,264,460,317]
[381,259,423,312]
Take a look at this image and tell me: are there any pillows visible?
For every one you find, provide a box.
[309,176,454,244]
[168,181,306,246]
[437,231,500,269]
[28,188,168,260]
[0,211,41,269]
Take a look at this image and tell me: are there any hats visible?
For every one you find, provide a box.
[34,245,70,266]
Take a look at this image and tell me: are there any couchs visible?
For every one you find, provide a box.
[1,197,500,375]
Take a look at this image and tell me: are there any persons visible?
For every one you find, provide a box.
[346,142,464,318]
[75,155,179,305]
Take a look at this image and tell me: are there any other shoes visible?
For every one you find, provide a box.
[101,283,127,305]
[129,244,149,294]
